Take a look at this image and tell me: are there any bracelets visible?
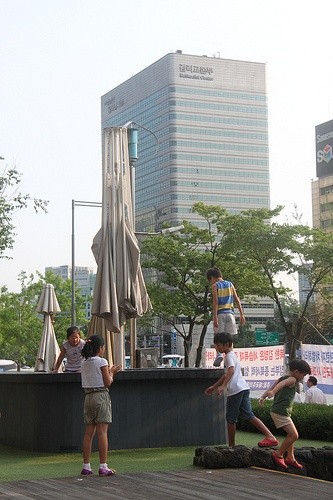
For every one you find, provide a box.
[216,381,221,386]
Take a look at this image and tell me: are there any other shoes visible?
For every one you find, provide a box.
[99,468,116,477]
[81,469,94,474]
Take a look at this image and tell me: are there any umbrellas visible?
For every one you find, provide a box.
[32,284,66,373]
[86,126,153,370]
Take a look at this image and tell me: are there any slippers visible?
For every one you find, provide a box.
[257,438,278,446]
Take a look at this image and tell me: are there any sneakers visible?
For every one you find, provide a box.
[213,353,223,367]
[285,457,303,469]
[271,451,288,471]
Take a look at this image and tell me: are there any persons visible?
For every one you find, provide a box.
[54,326,86,373]
[203,333,279,446]
[305,376,327,405]
[80,334,122,477]
[258,360,311,469]
[206,267,250,365]
